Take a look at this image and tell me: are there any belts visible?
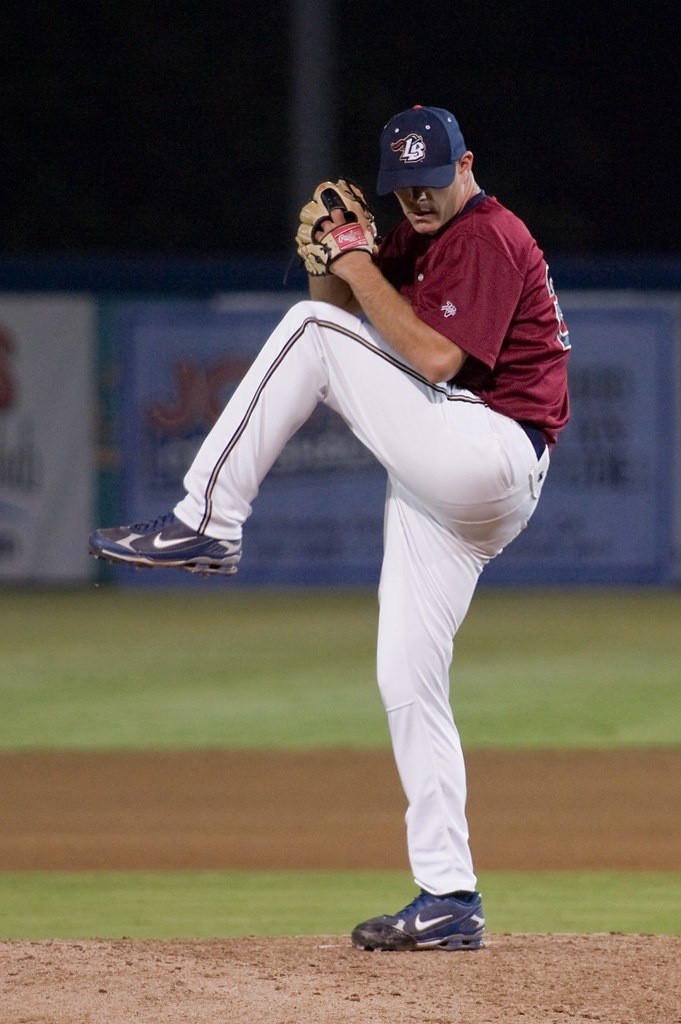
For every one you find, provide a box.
[516,420,545,479]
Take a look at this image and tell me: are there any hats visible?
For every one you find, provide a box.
[376,105,466,195]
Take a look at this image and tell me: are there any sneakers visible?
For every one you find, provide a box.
[351,888,485,951]
[88,513,242,577]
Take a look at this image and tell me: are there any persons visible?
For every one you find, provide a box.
[84,104,571,954]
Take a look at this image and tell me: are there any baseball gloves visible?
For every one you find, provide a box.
[295,177,381,277]
